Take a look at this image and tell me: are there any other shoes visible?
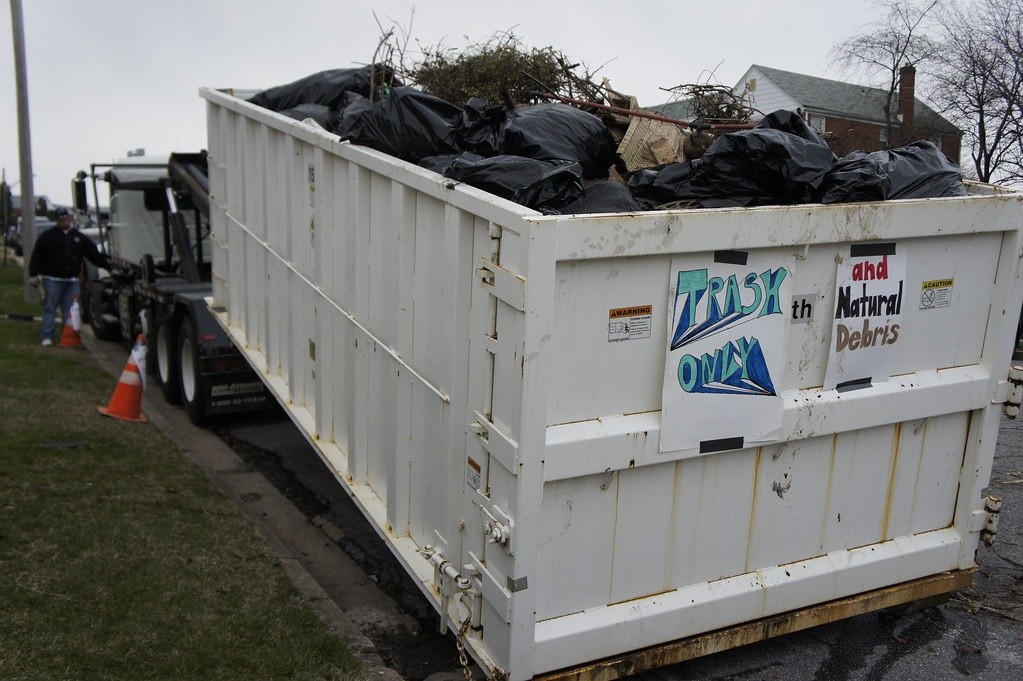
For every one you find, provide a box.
[42,339,53,346]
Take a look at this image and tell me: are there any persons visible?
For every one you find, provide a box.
[29,207,120,348]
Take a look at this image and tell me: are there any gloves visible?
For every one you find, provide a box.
[30,277,39,288]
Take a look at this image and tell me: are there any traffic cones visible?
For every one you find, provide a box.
[56,296,86,351]
[97,332,149,423]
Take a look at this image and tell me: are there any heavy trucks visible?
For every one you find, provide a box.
[73,85,1021,680]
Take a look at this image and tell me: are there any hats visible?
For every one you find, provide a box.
[54,208,69,221]
